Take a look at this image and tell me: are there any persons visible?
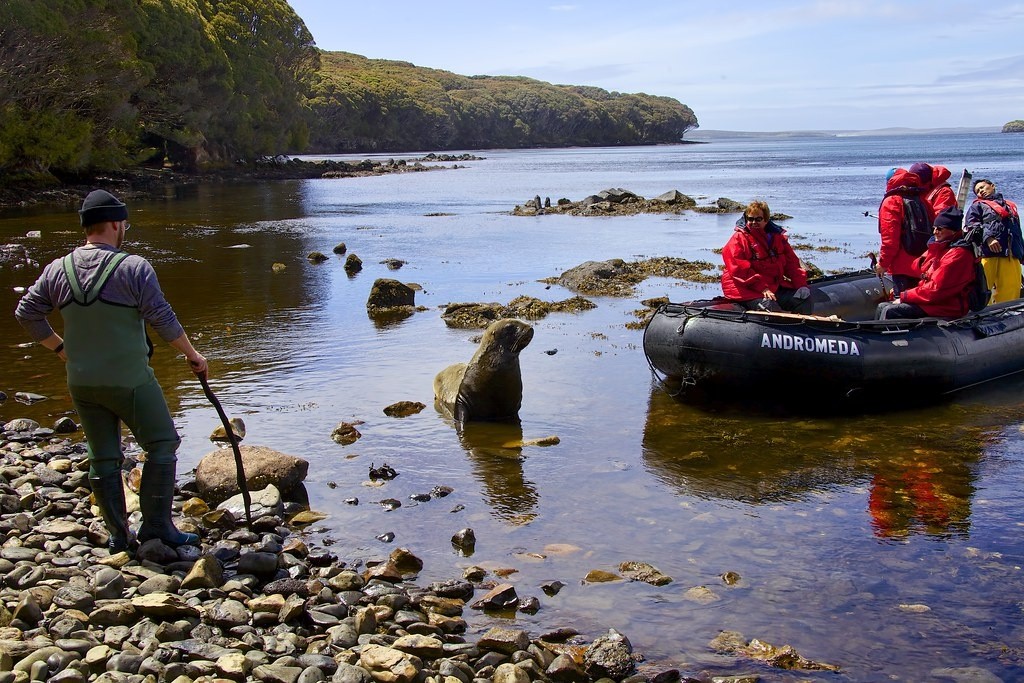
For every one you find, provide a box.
[721,199,813,321]
[875,160,1024,321]
[11,185,214,553]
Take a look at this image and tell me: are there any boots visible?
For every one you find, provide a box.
[138,461,201,547]
[88,468,136,555]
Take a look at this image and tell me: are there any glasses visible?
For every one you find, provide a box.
[932,226,943,231]
[745,216,764,221]
[125,223,131,231]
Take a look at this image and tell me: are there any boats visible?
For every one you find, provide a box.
[643,268,1024,412]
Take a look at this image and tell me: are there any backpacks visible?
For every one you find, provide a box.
[901,195,934,256]
[965,258,992,312]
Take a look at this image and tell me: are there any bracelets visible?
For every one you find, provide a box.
[54,342,64,354]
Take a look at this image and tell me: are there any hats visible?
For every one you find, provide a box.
[77,189,128,227]
[932,203,964,231]
[885,168,899,179]
[908,161,932,184]
